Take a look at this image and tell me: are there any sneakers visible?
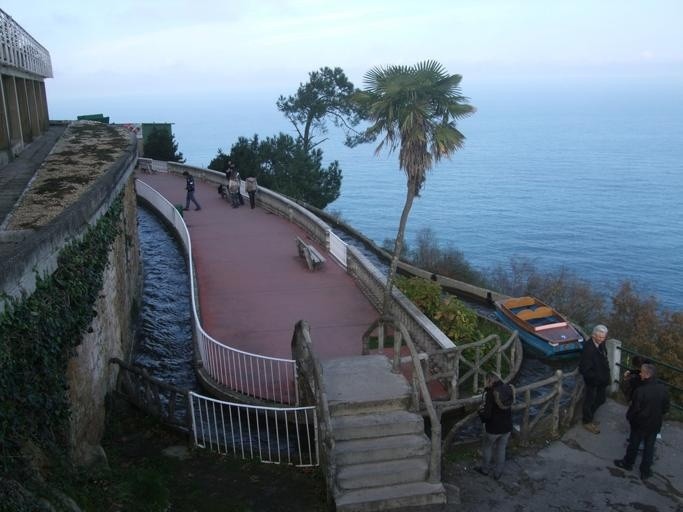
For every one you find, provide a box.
[479,466,490,476]
[613,458,634,472]
[493,473,501,481]
[641,469,653,480]
[585,418,601,434]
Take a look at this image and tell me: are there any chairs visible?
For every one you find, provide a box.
[505,296,553,320]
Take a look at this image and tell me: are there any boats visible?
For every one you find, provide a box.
[494,295,585,362]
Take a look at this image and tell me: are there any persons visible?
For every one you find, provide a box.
[614,364,670,480]
[473,372,515,481]
[577,324,612,435]
[218,157,260,209]
[181,170,201,211]
[619,354,649,452]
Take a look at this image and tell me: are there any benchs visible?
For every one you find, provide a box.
[295,234,326,271]
